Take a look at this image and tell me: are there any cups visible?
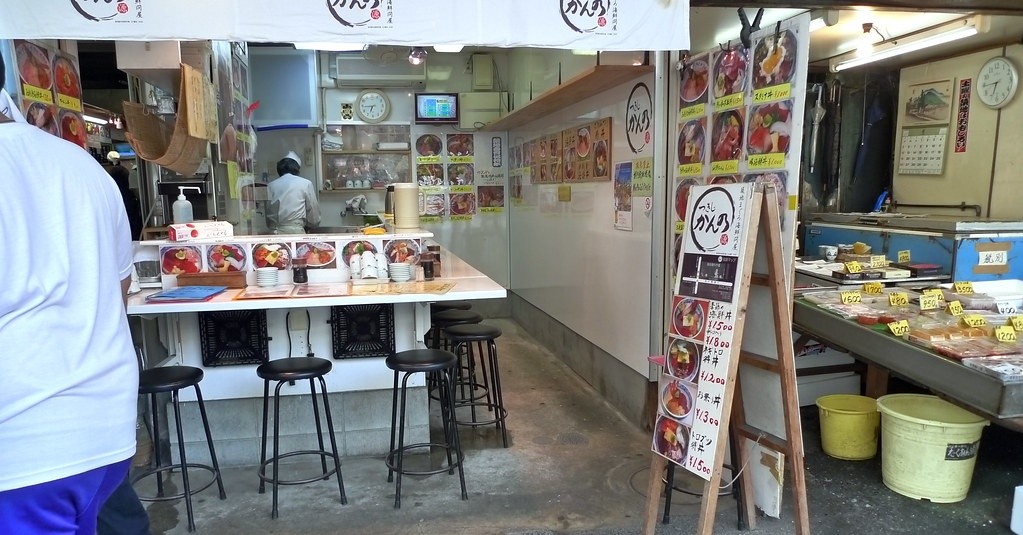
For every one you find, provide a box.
[420,259,434,281]
[825,246,838,260]
[346,180,371,188]
[349,251,388,279]
[818,245,828,259]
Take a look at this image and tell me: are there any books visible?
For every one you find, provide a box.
[145,286,228,305]
[235,283,354,298]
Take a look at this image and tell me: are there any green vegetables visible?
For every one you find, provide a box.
[355,243,366,253]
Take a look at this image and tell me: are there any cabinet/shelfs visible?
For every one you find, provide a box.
[319,120,415,194]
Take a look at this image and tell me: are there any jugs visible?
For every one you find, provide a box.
[391,182,427,229]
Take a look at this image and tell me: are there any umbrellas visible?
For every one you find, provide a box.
[823,79,842,190]
[810,82,827,173]
[854,83,885,182]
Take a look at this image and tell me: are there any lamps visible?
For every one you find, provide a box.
[809,10,841,33]
[827,15,992,76]
[408,45,428,65]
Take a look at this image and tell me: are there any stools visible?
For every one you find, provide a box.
[432,308,495,415]
[382,345,474,509]
[444,321,513,450]
[250,353,351,519]
[130,365,226,533]
[431,300,481,391]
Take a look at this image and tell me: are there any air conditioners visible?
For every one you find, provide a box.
[333,53,427,91]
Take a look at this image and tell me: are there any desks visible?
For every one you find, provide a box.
[424,238,489,278]
[128,277,505,313]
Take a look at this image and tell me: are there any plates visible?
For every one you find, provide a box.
[257,267,279,286]
[674,177,700,222]
[389,263,411,282]
[712,50,747,103]
[746,100,792,159]
[341,240,378,268]
[677,119,706,164]
[383,239,421,265]
[680,59,709,102]
[710,174,737,184]
[593,140,608,177]
[207,244,247,272]
[710,109,745,161]
[577,128,591,158]
[251,243,293,271]
[743,172,787,229]
[160,246,203,275]
[416,134,475,216]
[751,30,798,89]
[16,42,87,150]
[296,244,336,268]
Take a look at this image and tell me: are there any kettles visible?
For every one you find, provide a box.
[384,185,394,214]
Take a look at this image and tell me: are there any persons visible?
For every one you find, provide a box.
[0,52,139,535]
[264,150,323,235]
[107,151,135,241]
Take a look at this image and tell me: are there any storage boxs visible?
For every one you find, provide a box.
[457,92,509,130]
[832,270,862,279]
[174,271,247,289]
[168,217,235,240]
[845,253,870,261]
[471,54,494,90]
[940,277,1023,307]
[863,272,882,279]
[942,287,995,309]
[376,142,410,151]
[889,262,945,277]
[882,266,911,278]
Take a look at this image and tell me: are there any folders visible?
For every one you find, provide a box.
[145,286,229,301]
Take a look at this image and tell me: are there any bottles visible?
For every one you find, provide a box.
[426,245,441,277]
[883,200,891,213]
[291,258,309,286]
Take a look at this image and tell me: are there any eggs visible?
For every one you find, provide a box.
[760,46,786,79]
[175,252,185,260]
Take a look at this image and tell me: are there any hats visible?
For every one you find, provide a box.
[283,151,301,165]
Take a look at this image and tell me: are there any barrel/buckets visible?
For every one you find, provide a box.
[816,394,881,461]
[876,393,991,504]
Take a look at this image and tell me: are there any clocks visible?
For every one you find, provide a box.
[356,88,391,123]
[975,54,1020,111]
